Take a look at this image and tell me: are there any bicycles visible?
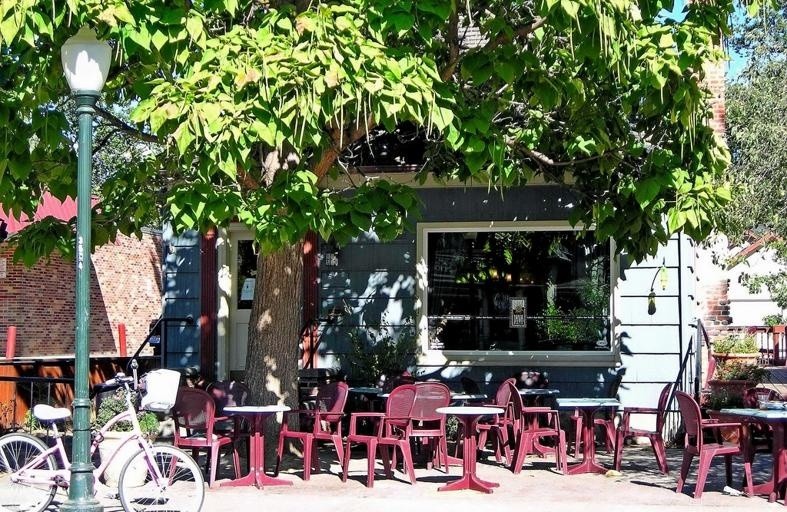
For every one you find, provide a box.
[1,357,206,512]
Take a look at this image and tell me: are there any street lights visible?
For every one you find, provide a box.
[59,26,114,511]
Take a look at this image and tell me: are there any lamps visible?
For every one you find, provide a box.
[649,253,668,293]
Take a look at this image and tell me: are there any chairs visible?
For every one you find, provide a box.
[168,386,240,489]
[178,370,254,472]
[509,381,567,473]
[343,384,418,487]
[274,381,349,481]
[614,381,672,474]
[296,369,516,472]
[570,407,615,457]
[674,392,753,497]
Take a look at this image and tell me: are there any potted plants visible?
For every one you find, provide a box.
[93,388,160,488]
[706,331,772,408]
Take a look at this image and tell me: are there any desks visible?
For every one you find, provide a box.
[436,407,506,495]
[556,398,623,474]
[219,405,293,490]
[707,407,784,498]
